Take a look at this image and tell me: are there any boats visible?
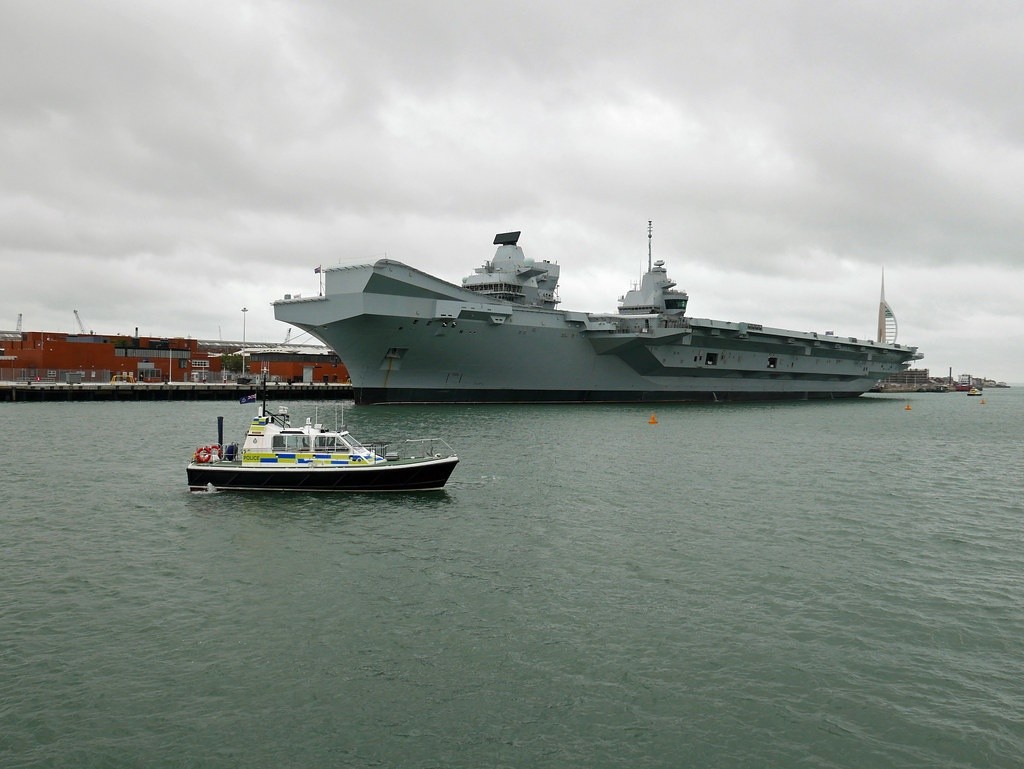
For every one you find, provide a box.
[185,367,462,494]
[967,386,983,396]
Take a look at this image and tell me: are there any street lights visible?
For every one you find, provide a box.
[241,307,249,378]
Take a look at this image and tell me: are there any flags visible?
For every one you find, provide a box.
[315,267,321,273]
[240,393,256,403]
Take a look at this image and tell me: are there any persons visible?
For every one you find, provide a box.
[140,373,143,381]
[203,375,206,383]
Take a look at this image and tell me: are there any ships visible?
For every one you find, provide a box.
[272,219,924,406]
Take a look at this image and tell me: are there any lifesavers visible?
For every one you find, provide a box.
[209,444,221,461]
[195,446,211,463]
